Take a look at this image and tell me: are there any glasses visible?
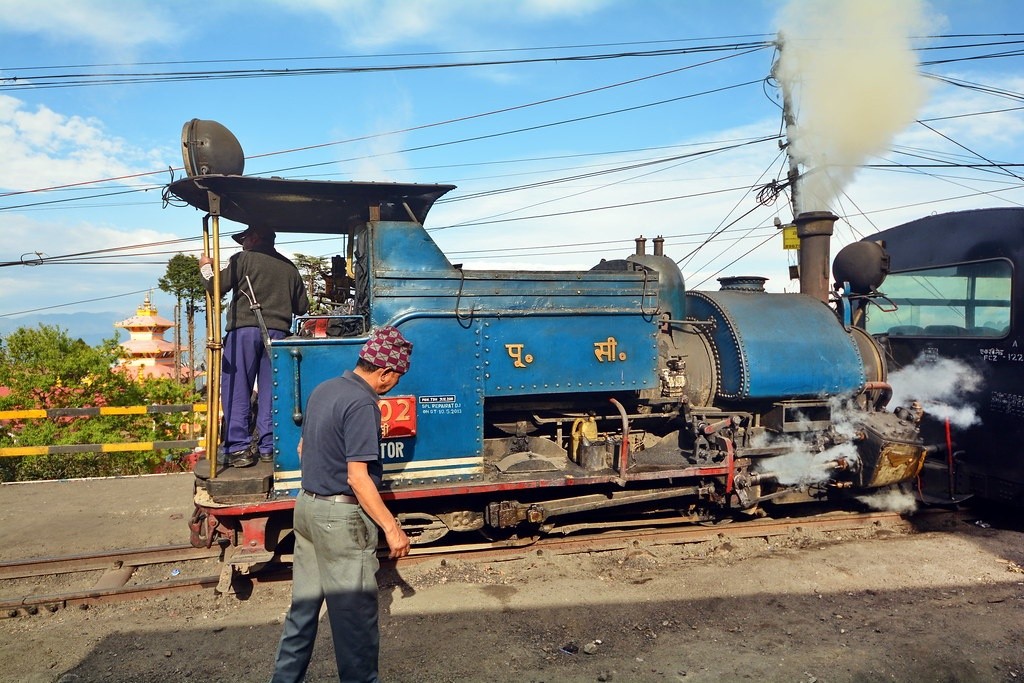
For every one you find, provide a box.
[239,232,251,244]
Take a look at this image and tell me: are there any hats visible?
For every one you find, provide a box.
[358,325,414,373]
[232,221,276,245]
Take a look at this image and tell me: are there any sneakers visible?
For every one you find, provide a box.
[216,446,254,467]
[257,446,273,461]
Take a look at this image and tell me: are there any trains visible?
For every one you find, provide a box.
[160,118,1024,593]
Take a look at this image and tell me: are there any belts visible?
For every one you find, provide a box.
[301,486,358,504]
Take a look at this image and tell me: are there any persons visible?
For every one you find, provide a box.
[270,326,413,683]
[199,223,310,467]
